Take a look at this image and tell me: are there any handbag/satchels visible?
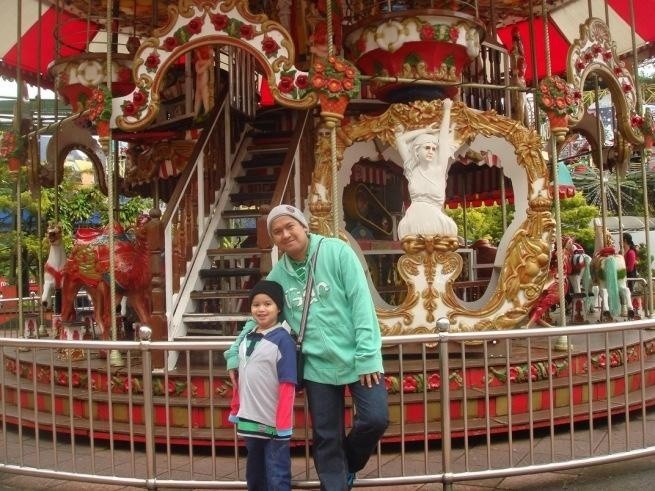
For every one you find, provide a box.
[291,334,303,388]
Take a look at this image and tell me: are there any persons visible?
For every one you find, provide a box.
[227,277,299,491]
[395,97,459,239]
[618,232,637,292]
[223,203,390,491]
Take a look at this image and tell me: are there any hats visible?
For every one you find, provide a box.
[249,280,282,311]
[267,204,309,238]
